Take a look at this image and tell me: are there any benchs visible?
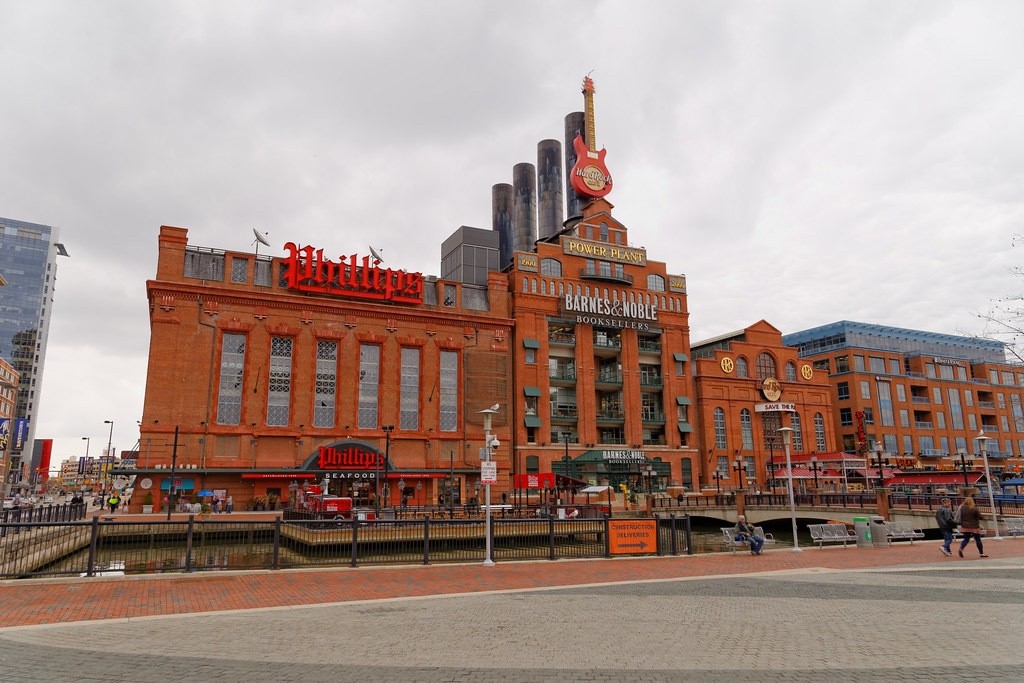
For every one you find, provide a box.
[807,523,858,550]
[952,524,987,542]
[1001,518,1024,539]
[720,527,775,555]
[885,521,925,546]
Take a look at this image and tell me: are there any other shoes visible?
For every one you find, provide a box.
[940,547,952,556]
[980,555,989,558]
[958,550,963,558]
[751,551,758,555]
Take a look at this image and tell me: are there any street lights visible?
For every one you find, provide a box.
[974,435,1004,539]
[950,448,975,487]
[809,456,823,488]
[100,420,114,510]
[561,431,572,476]
[82,437,89,479]
[475,409,497,566]
[398,478,406,520]
[380,425,394,507]
[767,437,776,494]
[712,467,725,494]
[777,426,801,552]
[416,482,423,518]
[867,443,891,487]
[732,455,749,489]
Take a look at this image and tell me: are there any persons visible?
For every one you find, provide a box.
[218,504,222,513]
[402,495,407,507]
[954,497,989,558]
[71,495,83,504]
[935,498,956,556]
[123,498,130,505]
[12,494,22,510]
[735,515,764,556]
[226,495,233,513]
[213,495,219,513]
[109,496,121,513]
[440,494,444,504]
[502,492,507,505]
[794,487,799,506]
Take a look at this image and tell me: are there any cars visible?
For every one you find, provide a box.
[65,491,111,506]
[125,487,134,497]
[2,494,55,512]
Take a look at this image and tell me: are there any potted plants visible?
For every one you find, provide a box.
[248,493,281,511]
[200,503,211,520]
[142,491,154,514]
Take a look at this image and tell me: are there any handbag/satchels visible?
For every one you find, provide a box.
[943,510,957,528]
[735,535,744,541]
[953,505,963,524]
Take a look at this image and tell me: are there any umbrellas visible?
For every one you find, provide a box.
[197,489,215,502]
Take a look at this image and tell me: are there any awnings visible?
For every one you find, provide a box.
[161,478,194,489]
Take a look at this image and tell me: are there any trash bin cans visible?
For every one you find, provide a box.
[869,516,889,546]
[854,516,873,547]
